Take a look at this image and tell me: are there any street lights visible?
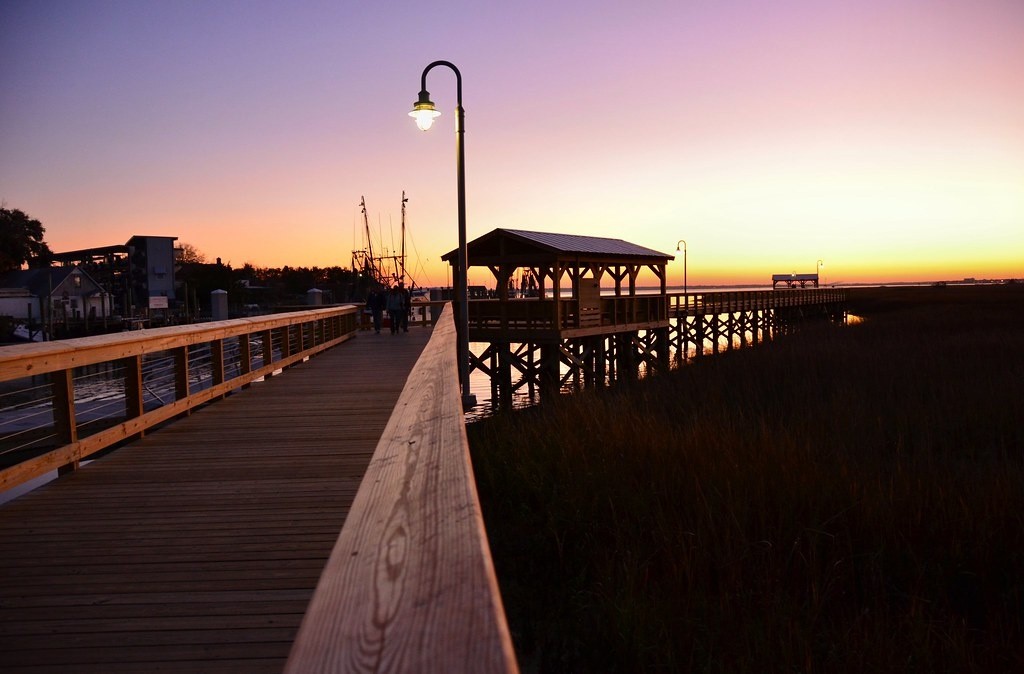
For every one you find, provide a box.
[409,59,472,395]
[816,260,823,290]
[791,270,798,288]
[675,239,687,294]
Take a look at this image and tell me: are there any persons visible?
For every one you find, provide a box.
[366,284,386,333]
[396,281,409,334]
[386,285,405,335]
